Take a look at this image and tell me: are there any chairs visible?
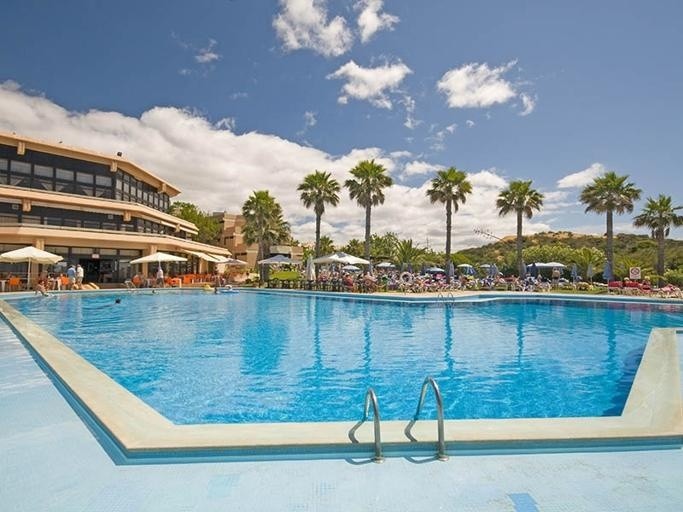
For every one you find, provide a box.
[259,276,347,291]
[0,271,79,293]
[398,271,475,293]
[124,274,213,289]
[593,279,682,300]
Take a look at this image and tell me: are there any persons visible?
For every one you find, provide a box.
[334,268,561,293]
[67,264,84,290]
[157,267,165,288]
[35,280,49,297]
[115,298,121,304]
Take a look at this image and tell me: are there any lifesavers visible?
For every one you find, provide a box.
[400,272,413,285]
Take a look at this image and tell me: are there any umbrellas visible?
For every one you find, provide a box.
[256,251,371,293]
[528,260,565,267]
[130,252,188,286]
[375,261,396,268]
[429,267,445,272]
[0,246,64,285]
[571,263,576,285]
[587,262,593,289]
[603,262,613,295]
[458,263,490,269]
